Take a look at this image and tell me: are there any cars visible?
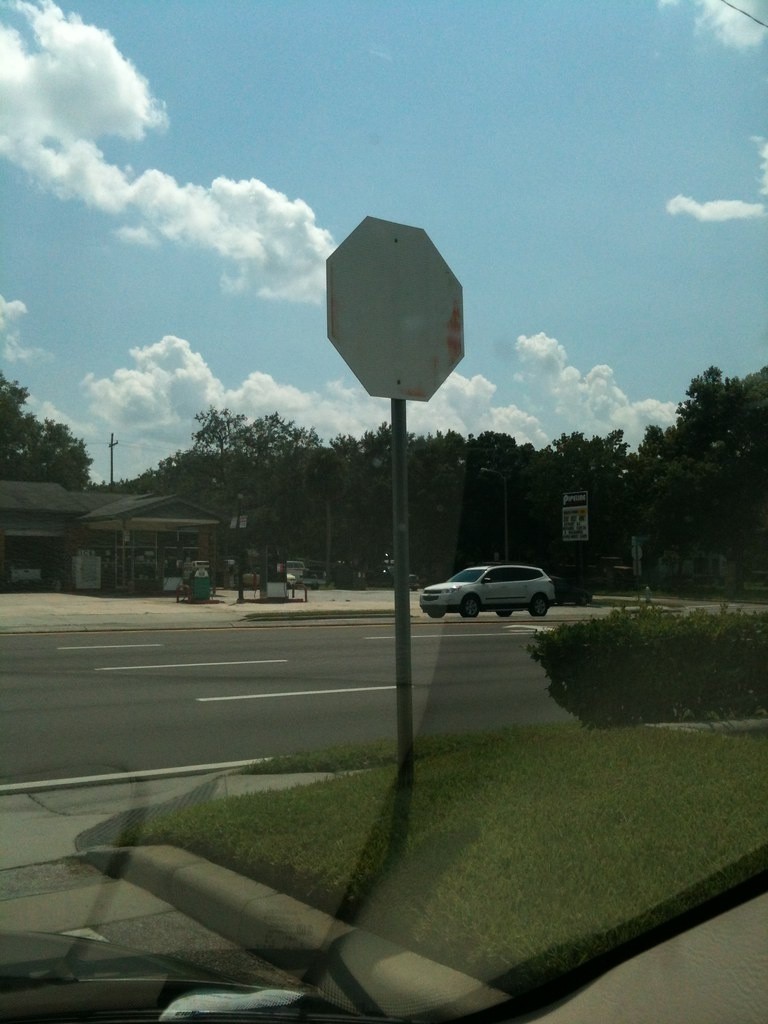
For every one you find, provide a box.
[243,557,420,591]
[4,558,65,594]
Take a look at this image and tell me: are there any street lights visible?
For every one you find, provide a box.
[478,467,509,562]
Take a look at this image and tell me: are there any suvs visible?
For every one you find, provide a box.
[417,564,557,618]
